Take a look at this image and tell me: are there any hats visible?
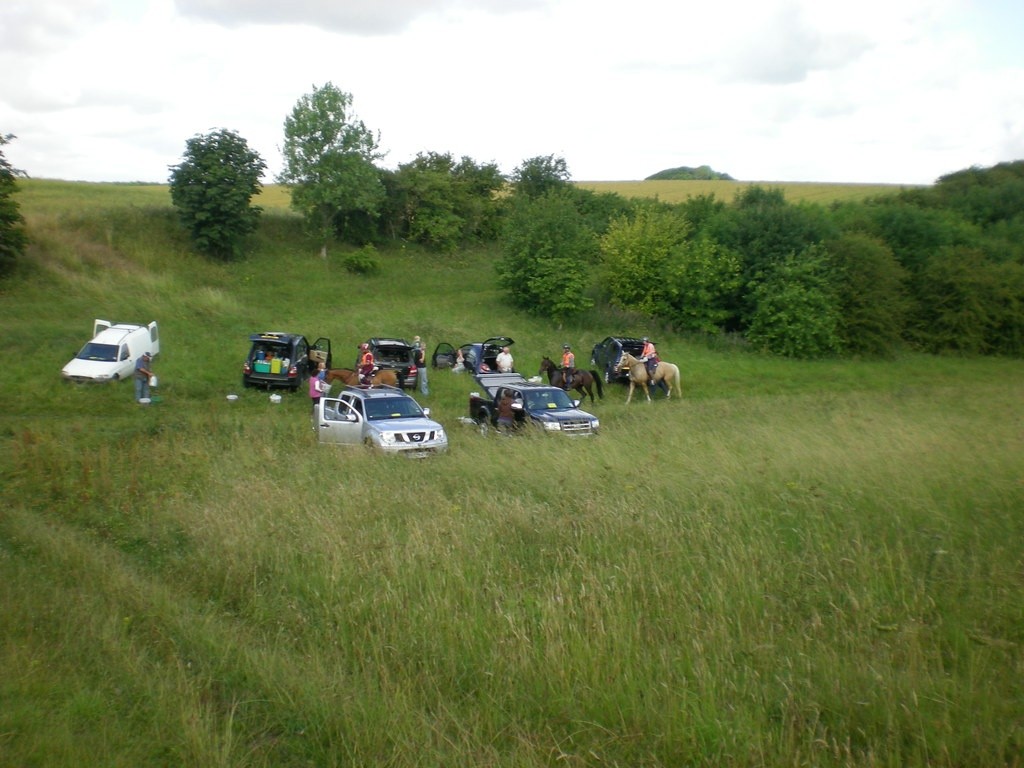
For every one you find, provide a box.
[144,352,152,360]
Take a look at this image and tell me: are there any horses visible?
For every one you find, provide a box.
[323,367,405,392]
[538,355,604,404]
[614,350,682,405]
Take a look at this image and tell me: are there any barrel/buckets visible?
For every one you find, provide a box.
[226,395,238,402]
[138,397,152,404]
[149,373,157,386]
[269,394,281,404]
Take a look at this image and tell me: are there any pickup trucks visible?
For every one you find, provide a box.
[468,382,600,438]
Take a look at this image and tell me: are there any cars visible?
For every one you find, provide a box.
[431,335,516,375]
[59,318,160,383]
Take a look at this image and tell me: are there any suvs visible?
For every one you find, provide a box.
[313,383,448,460]
[241,332,333,392]
[355,337,418,390]
[590,335,660,384]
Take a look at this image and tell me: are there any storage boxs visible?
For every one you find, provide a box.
[254,362,271,373]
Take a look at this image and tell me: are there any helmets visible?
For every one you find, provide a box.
[362,344,369,350]
[643,337,649,343]
[563,344,570,349]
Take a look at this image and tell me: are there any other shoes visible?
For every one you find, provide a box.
[650,379,655,386]
[566,386,572,390]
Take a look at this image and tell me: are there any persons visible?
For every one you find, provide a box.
[410,335,429,395]
[499,389,515,437]
[356,343,375,385]
[639,336,670,397]
[451,349,466,372]
[561,344,575,390]
[309,363,327,415]
[496,347,514,372]
[134,352,155,403]
